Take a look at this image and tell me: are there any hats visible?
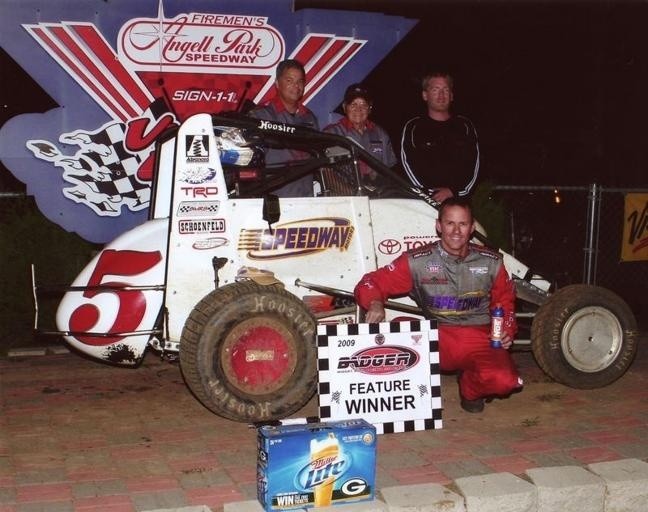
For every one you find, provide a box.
[344,83,368,104]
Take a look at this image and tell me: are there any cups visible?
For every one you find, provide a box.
[310,439,339,507]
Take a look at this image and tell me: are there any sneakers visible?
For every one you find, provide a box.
[461,398,483,413]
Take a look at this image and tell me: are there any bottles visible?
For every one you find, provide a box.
[490,302,504,348]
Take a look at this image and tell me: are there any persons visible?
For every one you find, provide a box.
[321,84,397,195]
[241,57,321,196]
[397,68,482,208]
[354,198,522,413]
